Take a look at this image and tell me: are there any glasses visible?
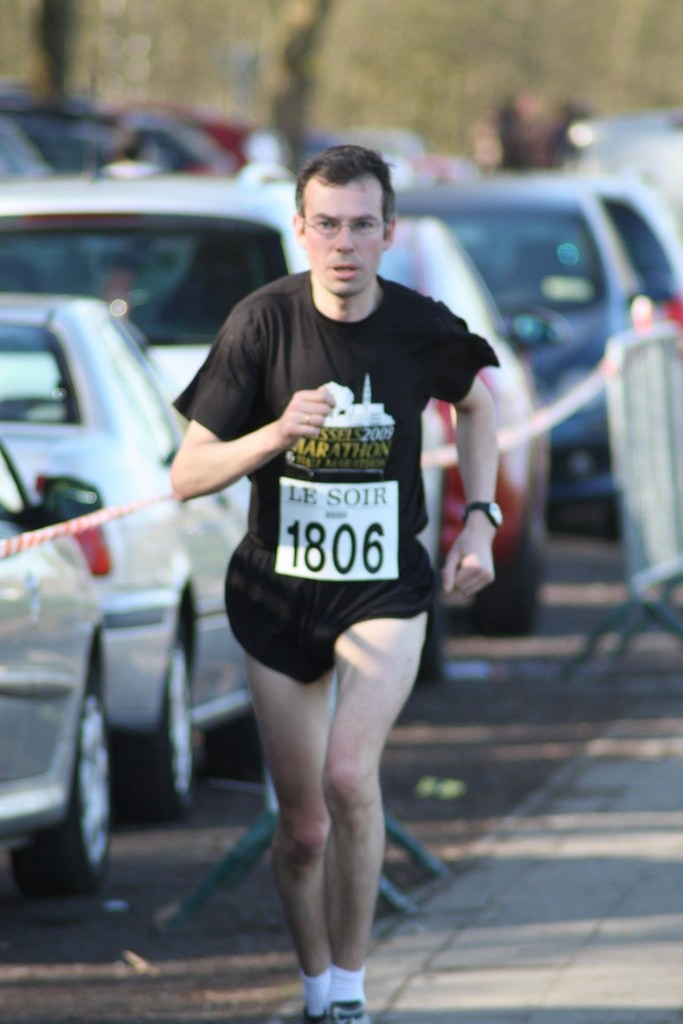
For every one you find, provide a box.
[302,218,385,235]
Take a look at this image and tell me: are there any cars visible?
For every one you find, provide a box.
[1,435,115,909]
[0,79,681,647]
[0,290,262,832]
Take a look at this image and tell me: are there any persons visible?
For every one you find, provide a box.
[169,143,505,1024]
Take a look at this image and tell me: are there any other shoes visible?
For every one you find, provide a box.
[328,1000,371,1024]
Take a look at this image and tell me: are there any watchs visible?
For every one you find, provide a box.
[462,500,503,528]
